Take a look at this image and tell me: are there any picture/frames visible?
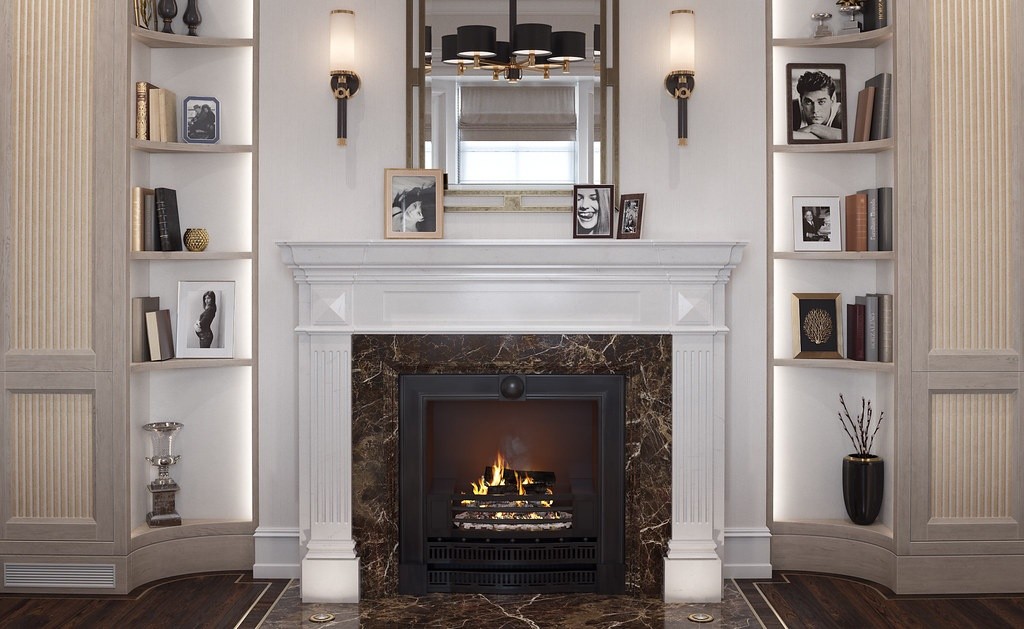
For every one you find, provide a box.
[134,0,158,30]
[383,168,444,239]
[792,293,845,359]
[180,95,220,144]
[792,196,841,252]
[787,62,848,144]
[572,184,615,239]
[174,280,236,359]
[617,194,646,239]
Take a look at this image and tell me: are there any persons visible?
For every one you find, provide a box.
[188,104,215,137]
[625,202,637,232]
[793,72,842,140]
[195,292,217,348]
[392,184,436,232]
[803,210,818,241]
[577,189,609,234]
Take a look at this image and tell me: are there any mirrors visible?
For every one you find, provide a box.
[406,0,620,214]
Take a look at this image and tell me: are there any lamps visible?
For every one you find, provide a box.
[664,10,696,145]
[331,10,360,144]
[442,0,587,84]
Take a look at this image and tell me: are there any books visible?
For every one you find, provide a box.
[845,187,892,251]
[863,0,886,32]
[847,293,892,362]
[133,296,174,362]
[133,186,183,251]
[137,81,178,143]
[853,73,891,142]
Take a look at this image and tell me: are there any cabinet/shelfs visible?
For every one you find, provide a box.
[765,0,899,595]
[129,0,256,595]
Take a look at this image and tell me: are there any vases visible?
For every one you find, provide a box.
[843,455,885,526]
